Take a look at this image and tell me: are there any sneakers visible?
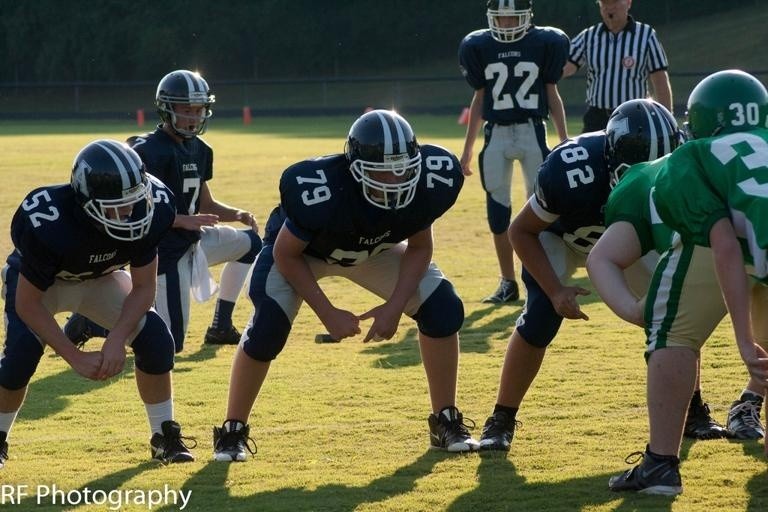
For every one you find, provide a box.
[0,431,9,468]
[150,420,193,461]
[727,392,764,441]
[213,419,249,461]
[483,276,520,303]
[479,411,515,450]
[683,389,726,438]
[428,406,479,454]
[204,324,242,344]
[608,443,683,497]
[63,314,90,347]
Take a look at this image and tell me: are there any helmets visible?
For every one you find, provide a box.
[684,68,768,139]
[487,0,534,42]
[156,69,216,138]
[603,100,680,189]
[345,109,423,211]
[71,139,156,241]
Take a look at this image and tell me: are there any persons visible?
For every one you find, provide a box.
[0,139,198,465]
[60,69,263,353]
[652,67,768,388]
[726,377,765,440]
[586,152,768,496]
[457,0,571,304]
[481,98,729,450]
[212,109,481,461]
[563,0,673,133]
